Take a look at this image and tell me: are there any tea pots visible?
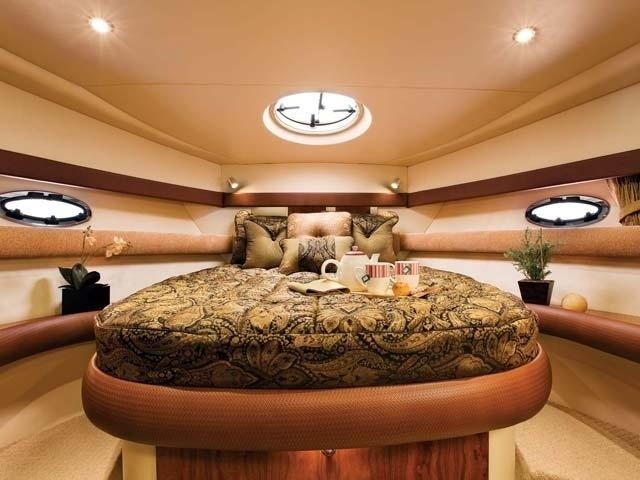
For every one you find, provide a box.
[320,245,380,292]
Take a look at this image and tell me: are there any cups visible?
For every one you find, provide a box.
[389,260,420,290]
[353,262,392,294]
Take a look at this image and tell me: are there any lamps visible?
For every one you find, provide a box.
[228,177,239,188]
[390,177,401,188]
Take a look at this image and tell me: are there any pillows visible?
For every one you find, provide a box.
[230,210,399,275]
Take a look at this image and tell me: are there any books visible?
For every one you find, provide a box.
[288,278,351,296]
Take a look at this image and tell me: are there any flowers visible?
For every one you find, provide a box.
[58,225,134,289]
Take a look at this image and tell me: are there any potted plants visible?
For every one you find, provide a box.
[504,225,555,305]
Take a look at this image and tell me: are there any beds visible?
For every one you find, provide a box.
[82,211,552,480]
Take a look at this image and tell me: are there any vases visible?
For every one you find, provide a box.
[62,289,110,315]
